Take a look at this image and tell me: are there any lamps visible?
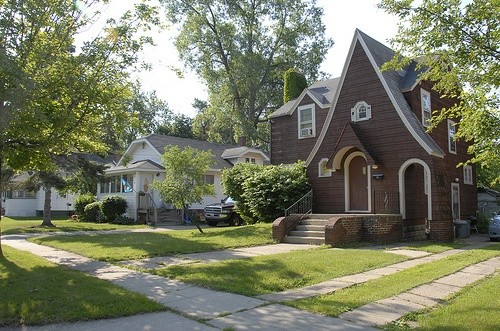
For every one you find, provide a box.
[157,173,161,177]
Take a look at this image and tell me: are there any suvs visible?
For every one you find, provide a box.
[203,194,244,227]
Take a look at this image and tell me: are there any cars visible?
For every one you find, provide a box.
[488,210,500,241]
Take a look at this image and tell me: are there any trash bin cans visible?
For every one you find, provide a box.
[454,219,471,238]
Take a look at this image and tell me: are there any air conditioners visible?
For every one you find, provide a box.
[301,128,312,137]
